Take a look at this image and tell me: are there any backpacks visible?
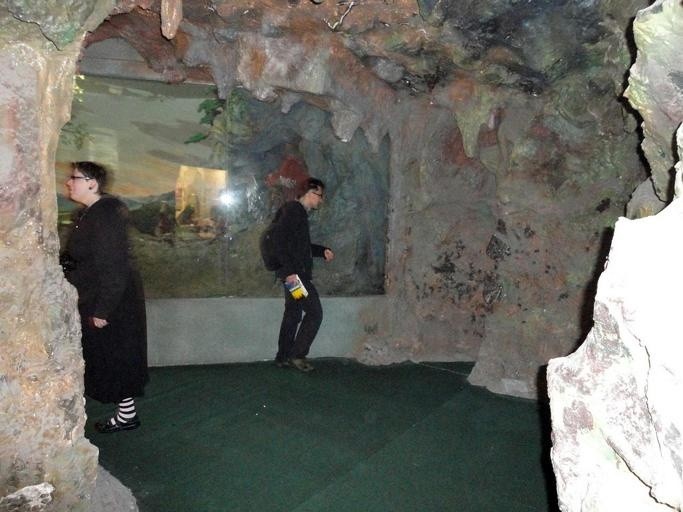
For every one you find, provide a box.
[259,206,296,272]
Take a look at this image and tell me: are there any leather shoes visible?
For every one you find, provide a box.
[95,416,140,433]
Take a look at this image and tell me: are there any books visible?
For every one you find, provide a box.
[283,274,308,304]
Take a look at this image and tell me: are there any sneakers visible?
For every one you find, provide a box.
[272,357,313,373]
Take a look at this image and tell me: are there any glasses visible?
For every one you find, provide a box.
[70,175,94,180]
[312,192,323,198]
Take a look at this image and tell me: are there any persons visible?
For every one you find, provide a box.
[61,161,148,433]
[270,177,334,372]
